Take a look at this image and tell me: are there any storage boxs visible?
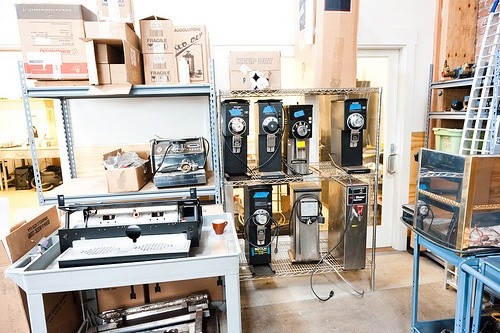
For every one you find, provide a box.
[432,128,484,156]
[103,149,150,193]
[15,4,210,95]
[228,50,282,90]
[0,205,82,333]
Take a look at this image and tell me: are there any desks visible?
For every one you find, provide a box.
[4,211,242,333]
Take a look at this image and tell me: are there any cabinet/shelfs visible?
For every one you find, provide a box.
[0,45,62,197]
[18,59,225,206]
[410,233,500,333]
[219,87,383,292]
[424,65,500,156]
